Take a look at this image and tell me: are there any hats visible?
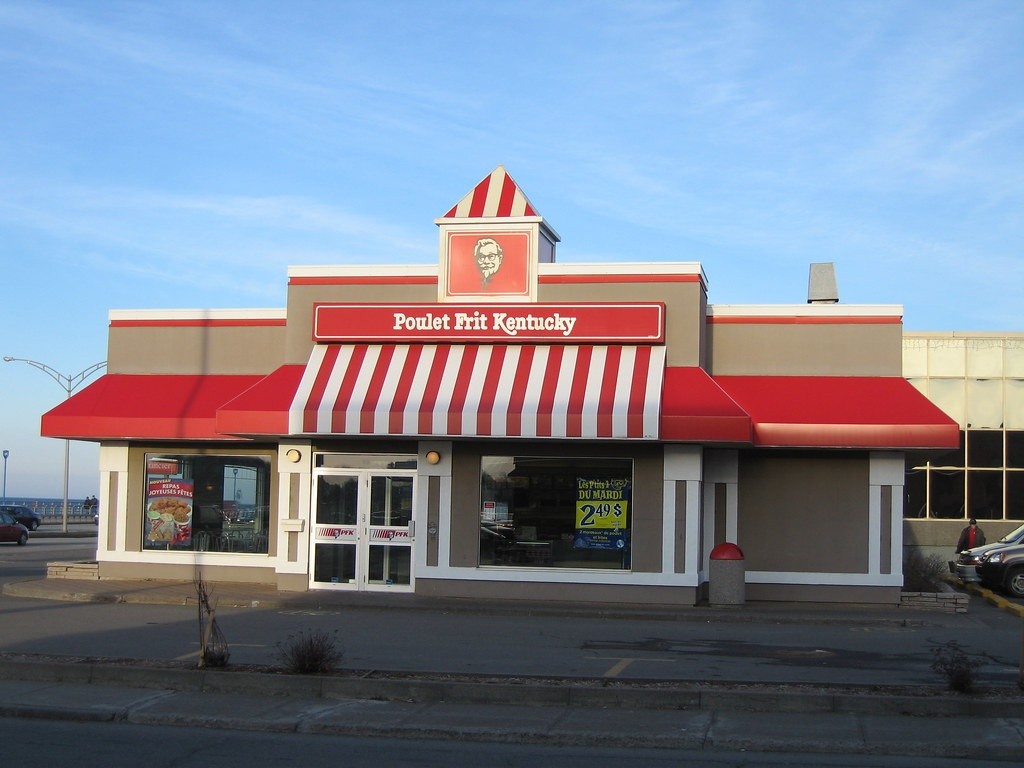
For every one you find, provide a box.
[969,518,976,524]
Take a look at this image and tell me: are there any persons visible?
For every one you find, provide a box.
[954,517,987,554]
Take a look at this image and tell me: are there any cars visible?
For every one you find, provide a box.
[0,511,30,547]
[956,523,1024,582]
[0,505,42,531]
[975,544,1024,597]
[480,519,515,551]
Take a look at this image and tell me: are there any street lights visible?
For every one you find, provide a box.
[2,449,9,506]
[232,469,240,510]
[4,353,111,534]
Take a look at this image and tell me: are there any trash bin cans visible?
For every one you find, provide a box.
[708,542,745,605]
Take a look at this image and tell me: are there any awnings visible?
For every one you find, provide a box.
[41,342,965,465]
